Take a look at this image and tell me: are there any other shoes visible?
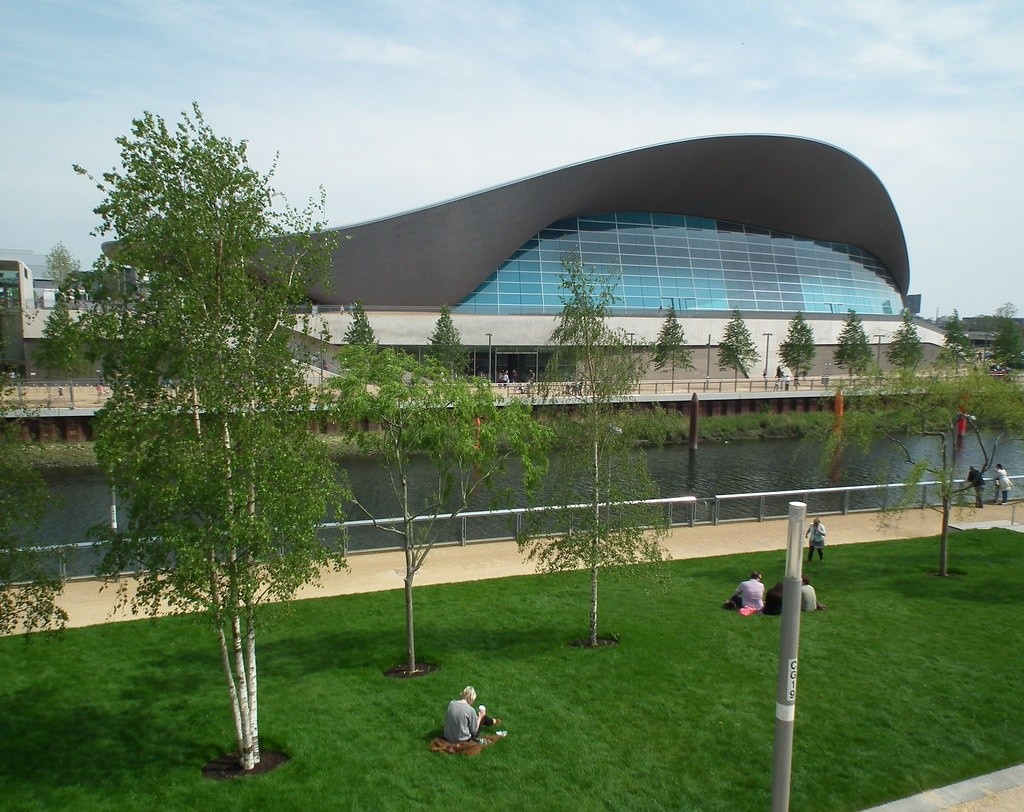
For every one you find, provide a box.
[496,719,501,725]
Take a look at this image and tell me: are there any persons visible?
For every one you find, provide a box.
[763,582,784,615]
[479,368,535,388]
[774,376,779,391]
[784,375,789,391]
[967,466,985,507]
[801,575,826,612]
[805,516,825,562]
[731,571,765,611]
[443,685,502,742]
[793,370,801,386]
[993,463,1014,504]
[94,369,109,404]
[775,365,781,378]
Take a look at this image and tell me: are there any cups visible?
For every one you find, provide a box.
[479,705,486,715]
